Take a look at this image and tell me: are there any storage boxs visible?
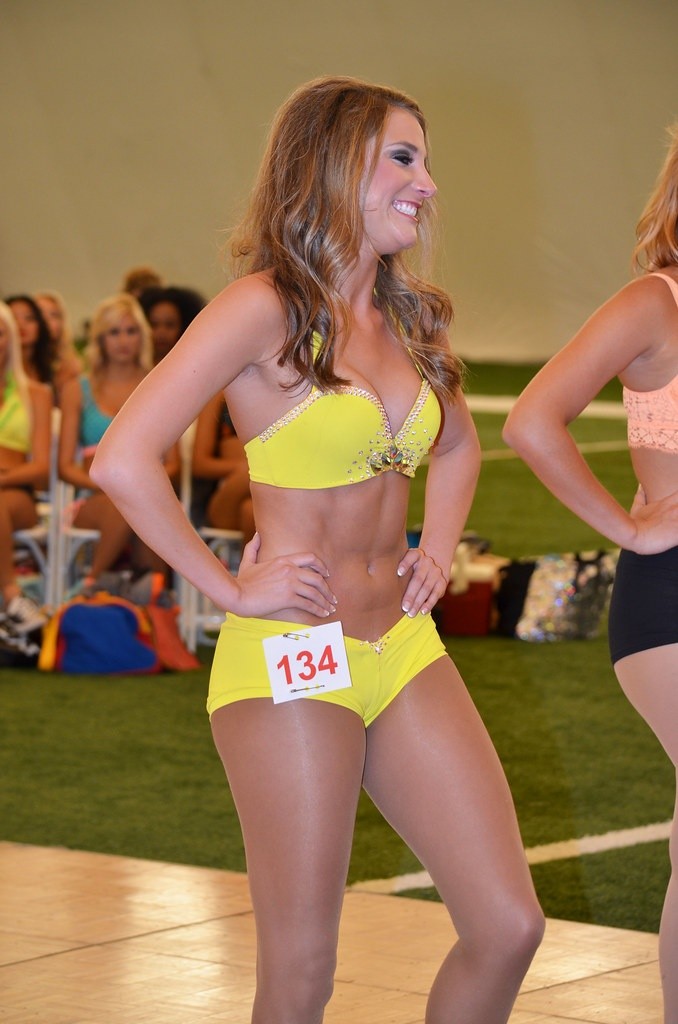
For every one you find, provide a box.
[440,542,495,636]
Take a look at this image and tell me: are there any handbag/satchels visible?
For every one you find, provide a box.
[37,572,201,673]
[493,550,618,640]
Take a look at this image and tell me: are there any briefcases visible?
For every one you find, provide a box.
[436,538,508,636]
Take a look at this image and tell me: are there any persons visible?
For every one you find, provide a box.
[501,129,678,1023]
[0,269,256,660]
[88,76,547,1024]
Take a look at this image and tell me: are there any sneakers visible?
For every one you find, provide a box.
[0,596,46,655]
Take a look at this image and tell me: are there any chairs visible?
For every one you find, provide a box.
[12,408,243,656]
